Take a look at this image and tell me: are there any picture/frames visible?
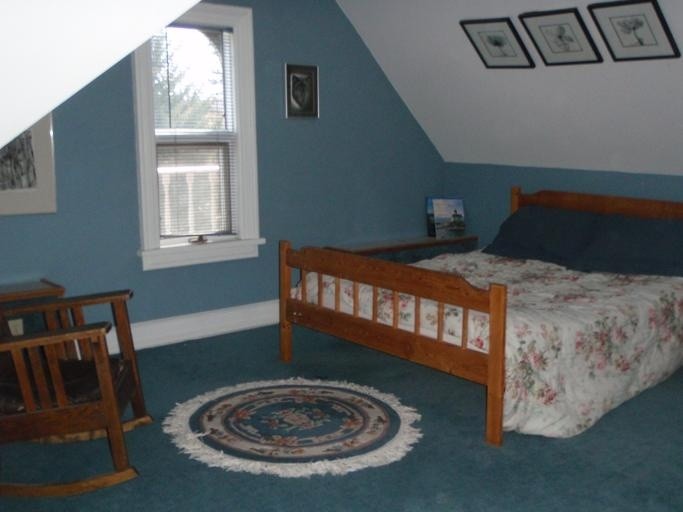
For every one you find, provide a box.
[458,0,681,67]
[0,110,57,216]
[284,64,319,118]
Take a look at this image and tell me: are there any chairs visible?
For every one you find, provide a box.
[0,289,156,498]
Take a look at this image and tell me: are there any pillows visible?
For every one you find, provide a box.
[481,202,682,277]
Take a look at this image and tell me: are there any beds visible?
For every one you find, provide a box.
[278,184,681,447]
